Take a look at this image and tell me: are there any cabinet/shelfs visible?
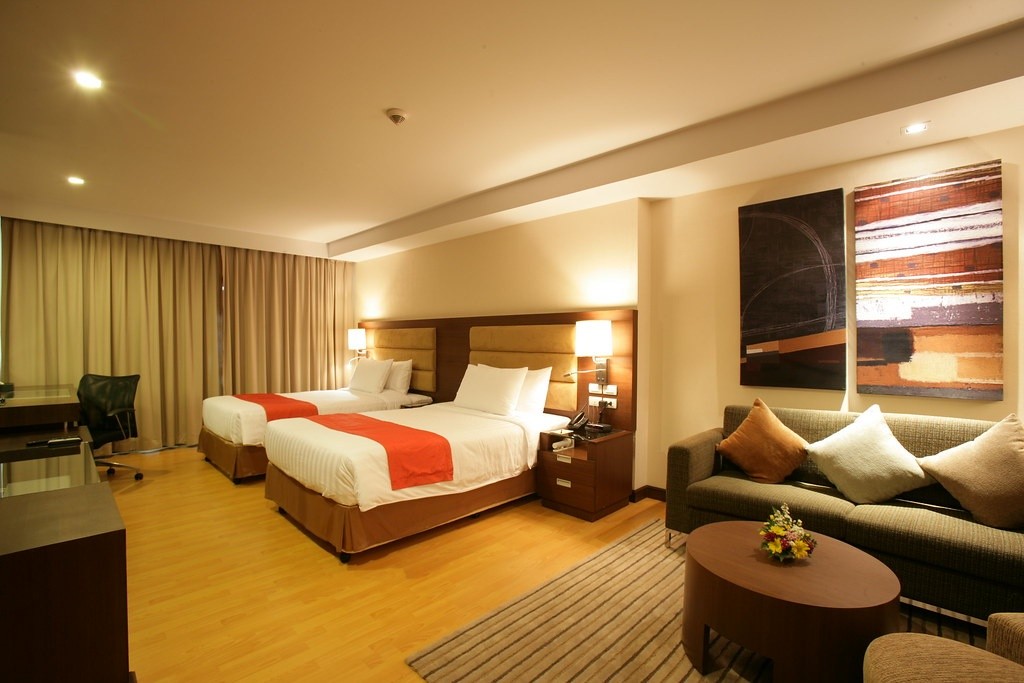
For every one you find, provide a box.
[0,425,137,683]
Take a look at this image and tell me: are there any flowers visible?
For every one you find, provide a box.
[759,502,817,561]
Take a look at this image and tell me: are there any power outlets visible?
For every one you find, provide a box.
[603,398,618,409]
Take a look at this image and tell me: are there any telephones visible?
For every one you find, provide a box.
[567,409,589,431]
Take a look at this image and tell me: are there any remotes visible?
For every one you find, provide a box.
[27,438,63,447]
[47,437,83,446]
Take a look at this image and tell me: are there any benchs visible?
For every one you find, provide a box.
[665,405,1023,622]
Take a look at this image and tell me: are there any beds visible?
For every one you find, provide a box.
[198,310,638,564]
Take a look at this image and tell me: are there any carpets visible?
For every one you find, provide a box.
[405,516,987,682]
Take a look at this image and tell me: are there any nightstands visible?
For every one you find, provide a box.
[538,430,633,522]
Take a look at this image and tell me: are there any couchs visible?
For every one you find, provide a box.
[864,613,1024,683]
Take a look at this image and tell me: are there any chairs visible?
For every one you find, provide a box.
[76,373,144,481]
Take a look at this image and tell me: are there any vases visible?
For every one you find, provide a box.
[773,554,795,563]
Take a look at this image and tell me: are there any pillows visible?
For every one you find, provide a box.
[476,364,552,416]
[915,413,1023,532]
[805,404,938,505]
[385,359,413,395]
[350,358,394,394]
[716,397,810,484]
[453,363,528,415]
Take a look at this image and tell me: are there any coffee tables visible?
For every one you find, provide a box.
[681,521,902,683]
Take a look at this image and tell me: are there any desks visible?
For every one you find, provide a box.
[0,384,81,432]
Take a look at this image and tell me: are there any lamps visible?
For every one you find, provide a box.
[563,320,613,385]
[348,328,366,369]
